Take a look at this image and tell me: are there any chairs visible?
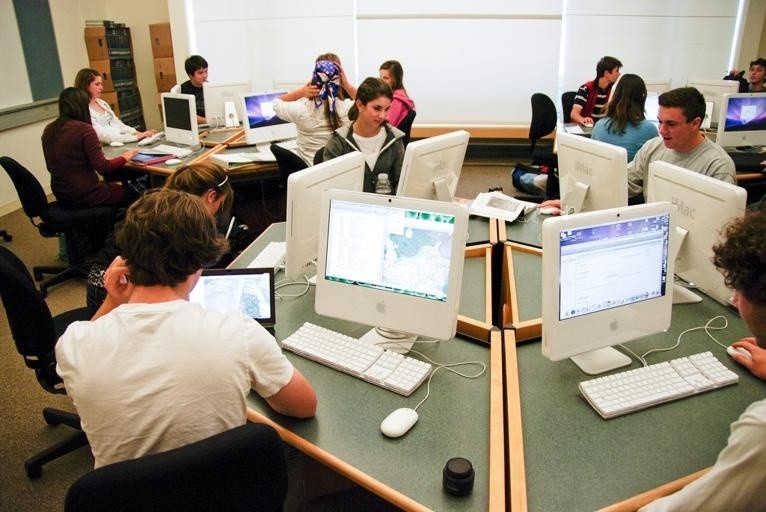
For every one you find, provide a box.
[526,91,558,168]
[562,91,578,124]
[3,99,416,296]
[2,246,161,480]
[64,423,288,510]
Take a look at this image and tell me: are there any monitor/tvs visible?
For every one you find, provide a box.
[637,80,766,147]
[396,128,471,205]
[314,185,469,356]
[555,132,628,214]
[541,200,677,375]
[284,150,366,286]
[644,159,748,307]
[161,80,307,151]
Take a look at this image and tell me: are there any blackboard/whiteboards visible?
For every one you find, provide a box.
[0,0,66,115]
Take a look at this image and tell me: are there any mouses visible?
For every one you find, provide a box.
[164,158,181,165]
[584,123,593,127]
[109,142,123,146]
[539,205,560,215]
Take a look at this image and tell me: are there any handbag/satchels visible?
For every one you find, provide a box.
[510,162,559,196]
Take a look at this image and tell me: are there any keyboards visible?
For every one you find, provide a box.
[137,132,164,145]
[151,144,192,158]
[245,241,285,276]
[280,321,432,397]
[577,350,740,421]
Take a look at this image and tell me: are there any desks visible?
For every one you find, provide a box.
[718,170,765,197]
[498,209,764,512]
[226,203,505,510]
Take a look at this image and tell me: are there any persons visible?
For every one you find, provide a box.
[75,68,158,144]
[86,161,230,317]
[273,53,359,167]
[379,61,414,127]
[323,78,406,196]
[628,88,737,199]
[41,87,140,207]
[181,55,209,124]
[55,189,317,471]
[724,59,766,92]
[591,74,658,164]
[570,56,623,126]
[635,210,765,511]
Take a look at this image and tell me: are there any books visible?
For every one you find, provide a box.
[465,190,536,223]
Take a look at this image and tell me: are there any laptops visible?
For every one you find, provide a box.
[188,268,275,337]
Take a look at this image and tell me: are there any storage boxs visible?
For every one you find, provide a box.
[84,26,146,133]
[148,23,177,130]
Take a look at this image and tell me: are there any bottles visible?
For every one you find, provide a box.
[442,456,476,497]
